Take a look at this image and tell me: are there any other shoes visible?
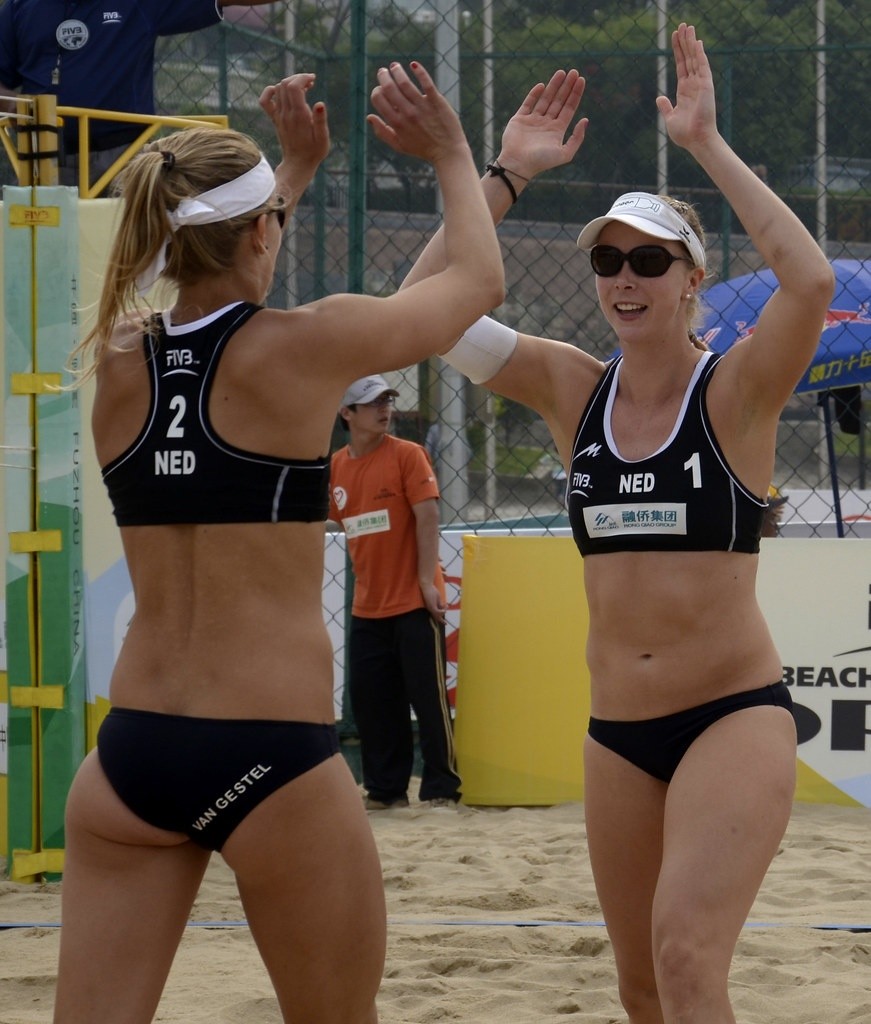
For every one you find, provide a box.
[429,798,457,814]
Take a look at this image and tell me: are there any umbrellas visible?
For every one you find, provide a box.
[617,257,870,538]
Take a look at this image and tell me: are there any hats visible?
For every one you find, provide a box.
[337,374,400,414]
[577,192,706,269]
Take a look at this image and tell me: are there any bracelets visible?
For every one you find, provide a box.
[484,159,529,203]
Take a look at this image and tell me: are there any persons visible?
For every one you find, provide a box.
[327,371,465,804]
[401,24,835,1023]
[0,0,277,202]
[55,61,508,1024]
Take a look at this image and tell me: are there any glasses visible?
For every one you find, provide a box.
[369,397,395,406]
[254,197,285,228]
[590,245,688,278]
